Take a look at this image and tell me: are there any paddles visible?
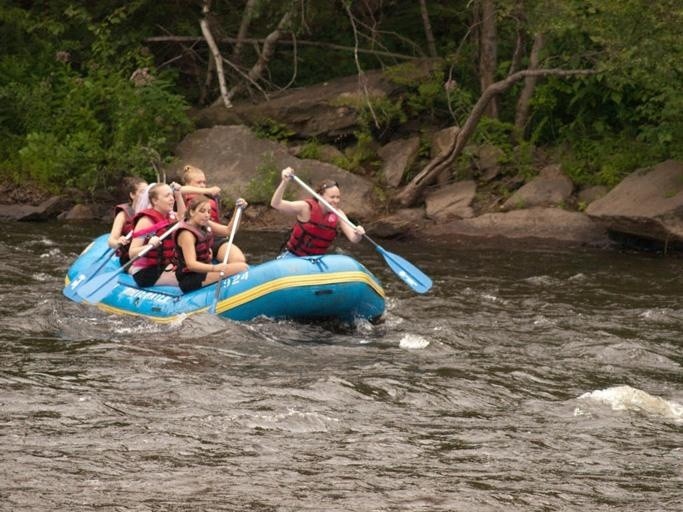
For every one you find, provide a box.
[76,216,186,304]
[62,229,135,304]
[284,169,432,297]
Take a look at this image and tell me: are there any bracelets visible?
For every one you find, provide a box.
[212,266,215,272]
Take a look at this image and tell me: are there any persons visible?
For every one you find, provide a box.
[271,167,365,260]
[108,176,152,273]
[172,195,248,293]
[173,165,246,262]
[128,182,186,286]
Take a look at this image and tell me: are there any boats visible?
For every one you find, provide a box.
[60,231,386,326]
